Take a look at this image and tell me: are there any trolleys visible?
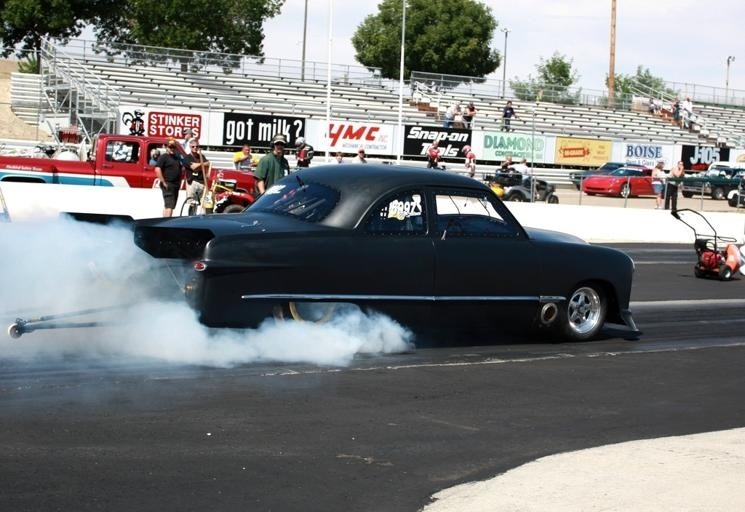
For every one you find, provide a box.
[670,207,743,281]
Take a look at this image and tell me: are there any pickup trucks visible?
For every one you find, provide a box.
[0,133,259,214]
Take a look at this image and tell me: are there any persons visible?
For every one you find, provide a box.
[648,97,692,129]
[443,102,462,129]
[334,152,346,164]
[351,149,368,164]
[665,161,685,211]
[426,141,440,169]
[148,129,314,219]
[461,144,476,178]
[502,155,538,197]
[500,101,520,132]
[461,102,477,129]
[652,162,667,209]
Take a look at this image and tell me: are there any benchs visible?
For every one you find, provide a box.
[423,92,745,147]
[49,58,438,127]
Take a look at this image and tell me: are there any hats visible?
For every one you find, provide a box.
[270,134,286,148]
[167,140,176,148]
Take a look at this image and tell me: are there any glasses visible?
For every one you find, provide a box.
[191,145,200,148]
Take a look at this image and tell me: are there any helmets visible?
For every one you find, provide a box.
[295,136,306,149]
[462,144,471,154]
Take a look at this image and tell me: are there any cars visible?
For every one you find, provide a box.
[481,161,745,210]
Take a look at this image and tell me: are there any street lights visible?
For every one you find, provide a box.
[726,57,736,104]
[396,1,411,165]
[500,27,511,96]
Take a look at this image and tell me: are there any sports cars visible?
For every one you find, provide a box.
[131,165,642,345]
